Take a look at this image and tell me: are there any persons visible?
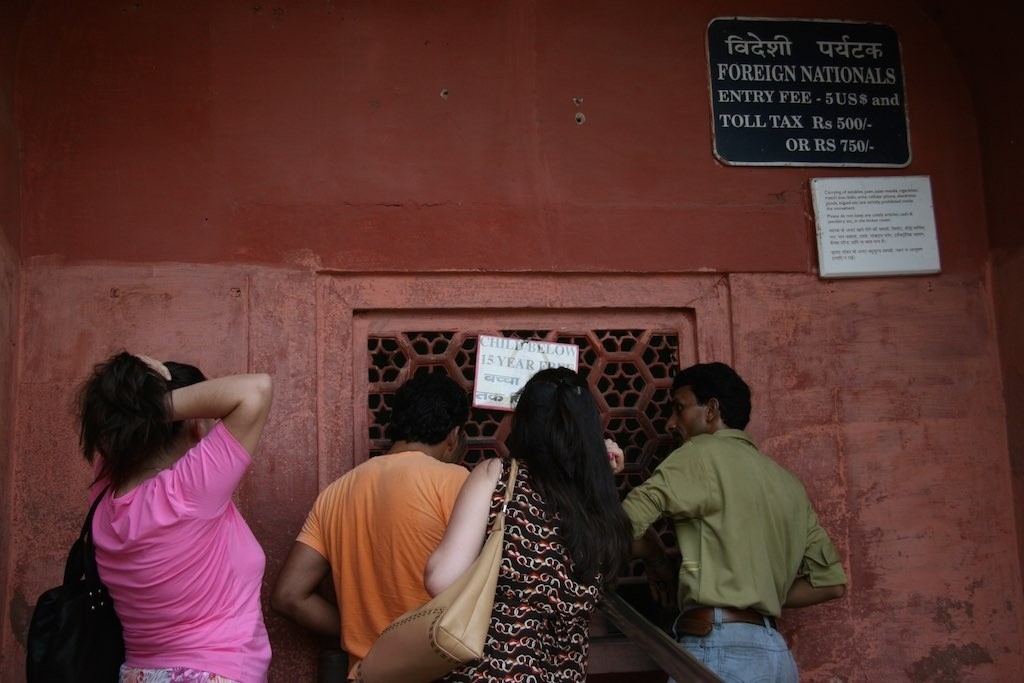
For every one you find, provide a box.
[70,348,275,683]
[269,373,471,683]
[620,361,847,683]
[424,364,625,683]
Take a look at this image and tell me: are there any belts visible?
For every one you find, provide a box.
[673,607,780,637]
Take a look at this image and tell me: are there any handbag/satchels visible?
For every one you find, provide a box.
[24,486,124,683]
[347,458,520,683]
